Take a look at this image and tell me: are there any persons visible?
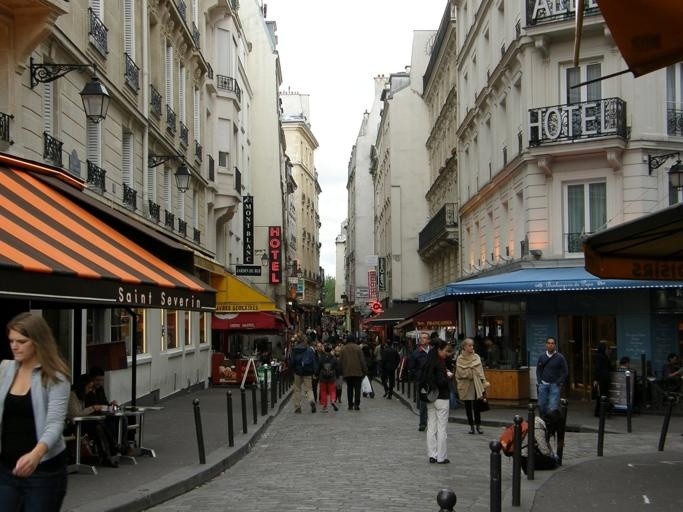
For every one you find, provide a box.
[0,312,74,512]
[618,356,644,409]
[536,338,568,413]
[505,409,565,476]
[418,340,451,465]
[401,332,499,434]
[663,355,683,391]
[79,368,143,457]
[58,374,120,462]
[593,343,611,418]
[274,322,401,414]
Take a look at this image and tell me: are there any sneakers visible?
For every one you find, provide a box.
[419,424,425,431]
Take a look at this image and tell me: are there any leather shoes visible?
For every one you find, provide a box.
[430,457,449,464]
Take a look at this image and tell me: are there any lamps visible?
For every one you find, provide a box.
[646,150,681,192]
[146,152,190,194]
[29,56,111,124]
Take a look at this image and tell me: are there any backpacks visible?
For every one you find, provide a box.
[499,421,545,457]
[322,363,335,379]
[418,382,438,402]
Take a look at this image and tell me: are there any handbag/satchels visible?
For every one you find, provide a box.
[478,397,489,412]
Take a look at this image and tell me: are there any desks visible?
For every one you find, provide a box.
[477,368,531,408]
[66,403,164,477]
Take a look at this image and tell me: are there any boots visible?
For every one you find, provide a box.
[469,424,483,434]
